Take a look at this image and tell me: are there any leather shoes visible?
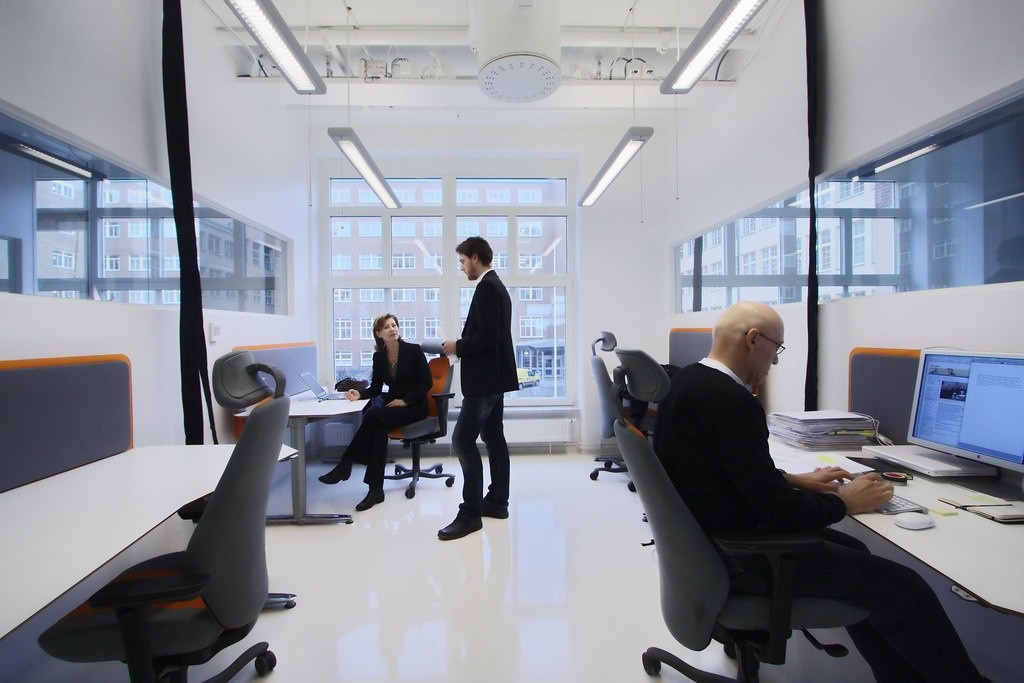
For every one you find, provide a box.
[438,511,483,540]
[459,500,509,518]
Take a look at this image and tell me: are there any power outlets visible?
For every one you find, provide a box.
[213,326,221,336]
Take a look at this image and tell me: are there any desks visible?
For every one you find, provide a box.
[0,354,298,683]
[766,347,1024,624]
[231,341,371,527]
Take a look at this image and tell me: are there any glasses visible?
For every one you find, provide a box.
[742,328,787,355]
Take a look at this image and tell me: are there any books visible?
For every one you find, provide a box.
[938,493,1024,522]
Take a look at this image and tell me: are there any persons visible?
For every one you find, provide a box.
[984,235,1024,285]
[317,313,434,512]
[654,299,995,683]
[439,237,521,542]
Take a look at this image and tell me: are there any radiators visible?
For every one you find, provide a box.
[388,418,577,456]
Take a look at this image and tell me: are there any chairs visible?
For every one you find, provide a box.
[589,331,851,683]
[36,338,459,683]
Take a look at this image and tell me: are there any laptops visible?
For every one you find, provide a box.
[299,371,350,401]
[861,444,998,477]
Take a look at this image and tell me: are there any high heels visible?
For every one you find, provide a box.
[318,456,353,485]
[356,485,384,511]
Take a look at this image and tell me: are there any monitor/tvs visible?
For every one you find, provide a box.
[907,348,1024,473]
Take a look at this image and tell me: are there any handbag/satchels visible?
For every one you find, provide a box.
[335,376,370,392]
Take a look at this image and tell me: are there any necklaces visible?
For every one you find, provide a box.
[387,348,398,368]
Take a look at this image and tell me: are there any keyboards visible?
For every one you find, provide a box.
[828,477,928,515]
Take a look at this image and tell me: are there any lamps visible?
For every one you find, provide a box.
[660,0,769,95]
[577,8,654,207]
[327,6,402,211]
[477,52,562,104]
[223,0,328,96]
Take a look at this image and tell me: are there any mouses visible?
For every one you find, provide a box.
[895,512,936,529]
